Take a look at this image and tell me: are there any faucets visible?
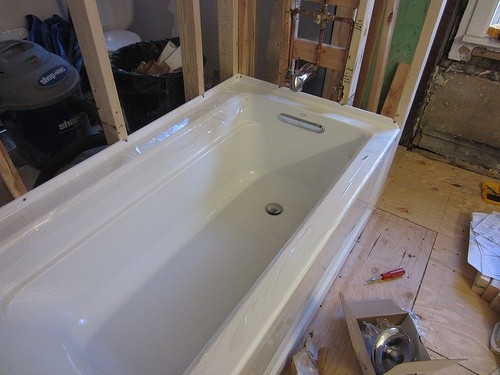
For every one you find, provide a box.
[290,63,317,90]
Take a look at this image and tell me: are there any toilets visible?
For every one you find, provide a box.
[91,0,143,54]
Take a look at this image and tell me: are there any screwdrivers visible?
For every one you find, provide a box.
[365,267,406,284]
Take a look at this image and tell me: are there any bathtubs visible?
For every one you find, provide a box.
[0,73,401,375]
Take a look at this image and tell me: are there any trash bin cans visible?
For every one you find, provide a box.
[108,33,206,132]
[0,40,95,171]
